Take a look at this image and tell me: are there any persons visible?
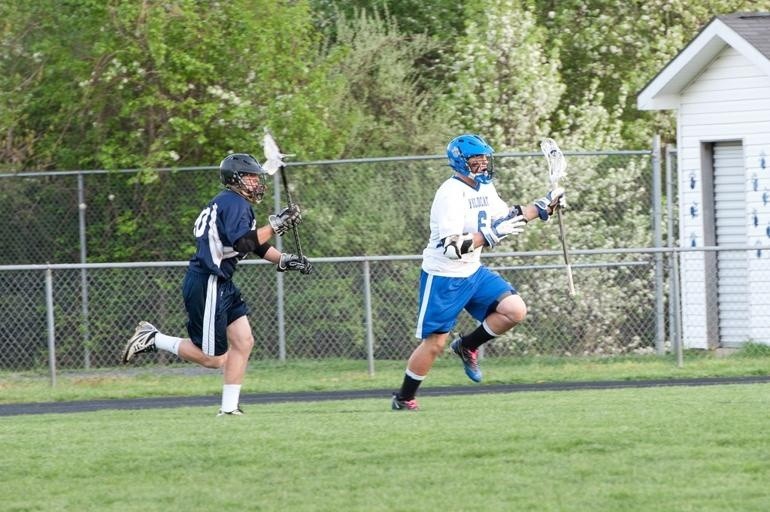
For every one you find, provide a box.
[391,134,566,413]
[121,153,312,417]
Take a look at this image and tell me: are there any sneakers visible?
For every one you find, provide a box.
[449,333,482,383]
[391,392,420,411]
[217,408,244,417]
[120,321,160,366]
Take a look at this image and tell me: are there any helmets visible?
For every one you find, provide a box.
[446,134,496,184]
[219,153,266,205]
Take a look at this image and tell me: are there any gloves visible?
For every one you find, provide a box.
[275,252,313,275]
[533,186,568,221]
[480,208,528,249]
[268,202,304,236]
